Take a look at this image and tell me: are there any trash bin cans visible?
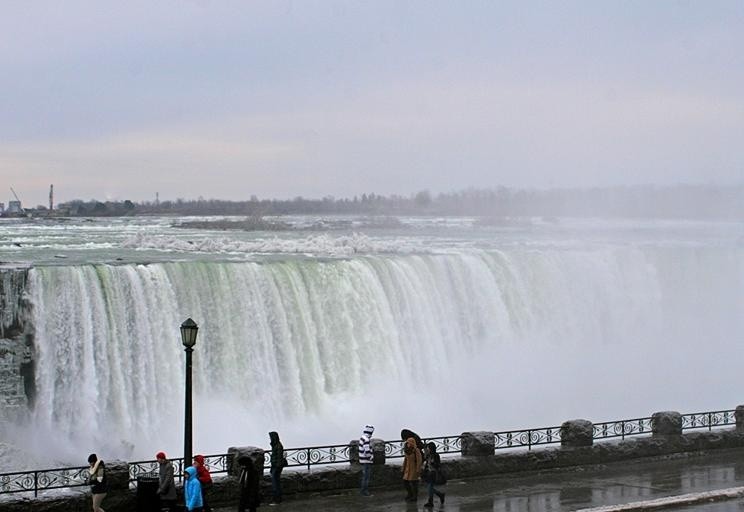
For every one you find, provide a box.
[137,476,160,512]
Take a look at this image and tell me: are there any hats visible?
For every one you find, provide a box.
[157,453,165,459]
[88,454,97,462]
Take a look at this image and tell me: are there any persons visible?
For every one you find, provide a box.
[88,454,107,512]
[184,466,203,512]
[238,456,259,512]
[194,455,212,512]
[401,429,445,507]
[359,426,374,498]
[156,452,177,512]
[269,432,283,506]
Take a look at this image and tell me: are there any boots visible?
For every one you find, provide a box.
[424,498,434,506]
[437,491,444,504]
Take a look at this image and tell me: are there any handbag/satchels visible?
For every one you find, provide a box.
[421,470,434,482]
[200,479,213,490]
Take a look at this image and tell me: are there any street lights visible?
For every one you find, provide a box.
[180,318,198,487]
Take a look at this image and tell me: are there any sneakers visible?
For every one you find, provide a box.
[405,495,417,502]
[361,492,373,497]
[269,500,283,505]
